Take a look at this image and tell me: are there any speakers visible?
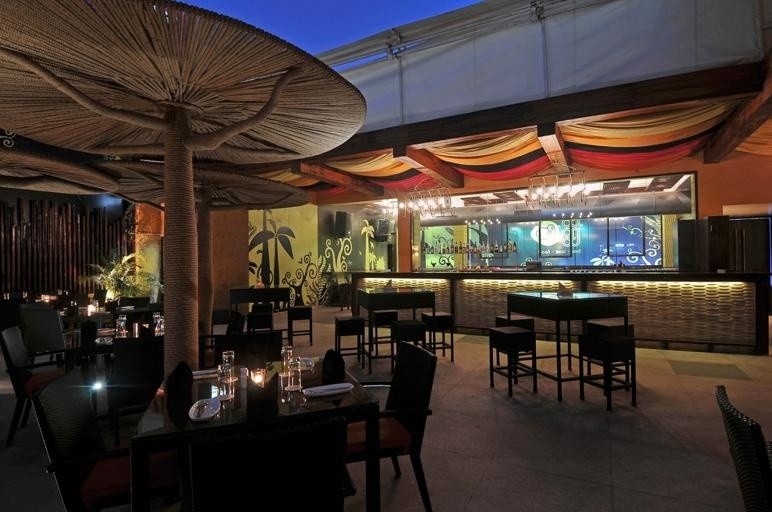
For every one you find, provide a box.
[673,219,696,269]
[376,219,389,241]
[699,216,729,274]
[336,211,352,237]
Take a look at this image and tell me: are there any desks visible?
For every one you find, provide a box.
[506,292,630,401]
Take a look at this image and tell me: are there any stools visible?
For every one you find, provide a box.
[421,310,454,362]
[489,315,637,411]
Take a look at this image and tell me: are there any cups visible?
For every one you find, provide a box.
[113,312,164,339]
[216,345,302,400]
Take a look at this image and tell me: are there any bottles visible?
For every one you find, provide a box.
[422,238,517,254]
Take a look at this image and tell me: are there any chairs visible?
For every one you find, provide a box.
[715,384,772,512]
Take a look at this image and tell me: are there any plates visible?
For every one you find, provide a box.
[187,396,221,423]
[299,383,354,398]
